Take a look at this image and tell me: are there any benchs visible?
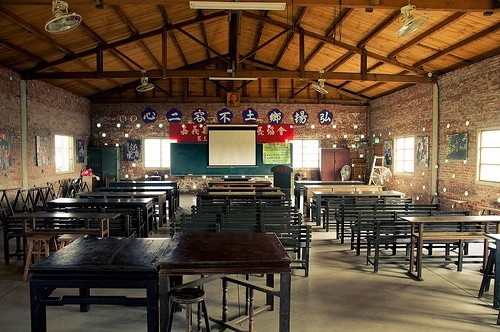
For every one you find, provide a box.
[4,193,497,299]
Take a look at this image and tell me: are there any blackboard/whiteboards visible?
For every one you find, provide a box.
[170,143,293,176]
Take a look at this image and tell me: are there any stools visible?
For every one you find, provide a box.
[168,287,212,332]
[23,232,58,281]
[57,234,85,248]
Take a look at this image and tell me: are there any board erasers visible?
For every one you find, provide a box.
[188,174,193,175]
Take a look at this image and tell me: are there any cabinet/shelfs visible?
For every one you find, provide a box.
[82,175,97,192]
[87,145,121,182]
[320,148,351,181]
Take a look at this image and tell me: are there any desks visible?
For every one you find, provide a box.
[94,186,174,218]
[4,211,121,266]
[46,196,157,237]
[294,180,365,209]
[206,186,281,192]
[303,184,386,218]
[196,191,286,199]
[76,190,167,227]
[29,233,159,332]
[311,190,403,226]
[109,180,180,207]
[159,231,293,332]
[208,180,272,187]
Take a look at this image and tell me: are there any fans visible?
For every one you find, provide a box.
[340,165,352,181]
[44,0,83,34]
[136,77,154,92]
[310,78,329,95]
[370,167,393,186]
[395,0,429,38]
